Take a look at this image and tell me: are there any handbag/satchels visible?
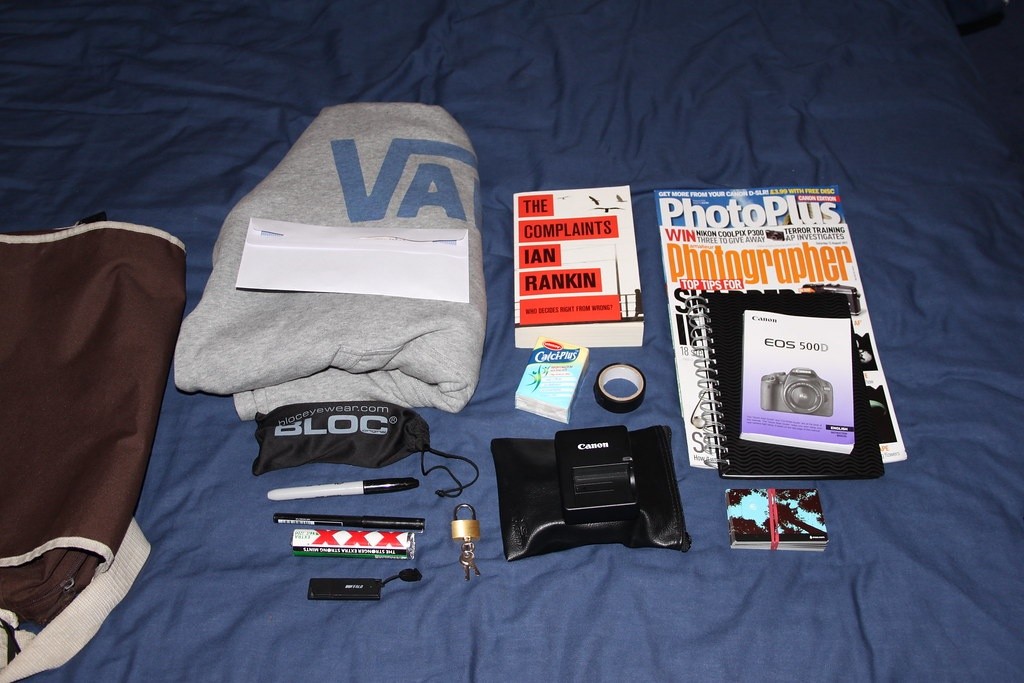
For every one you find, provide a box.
[0,212,186,683]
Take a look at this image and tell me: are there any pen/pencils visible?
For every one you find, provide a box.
[273,512,426,530]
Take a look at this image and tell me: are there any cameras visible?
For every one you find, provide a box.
[761,368,834,416]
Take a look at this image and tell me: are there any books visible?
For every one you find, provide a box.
[514,185,645,350]
[654,187,907,478]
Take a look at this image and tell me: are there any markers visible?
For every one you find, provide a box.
[267,477,420,501]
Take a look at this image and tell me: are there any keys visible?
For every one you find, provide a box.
[457,540,481,580]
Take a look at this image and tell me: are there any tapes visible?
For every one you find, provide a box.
[593,362,647,414]
